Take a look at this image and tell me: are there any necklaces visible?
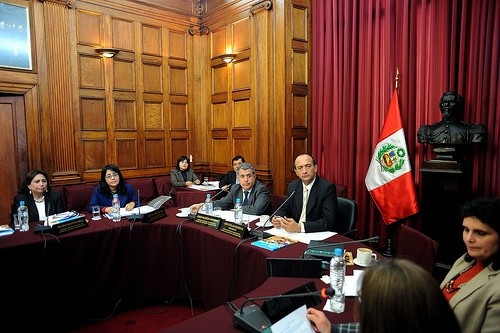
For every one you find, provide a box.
[34,196,44,203]
[446,265,473,294]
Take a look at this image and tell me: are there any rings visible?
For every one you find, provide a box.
[284,228,286,231]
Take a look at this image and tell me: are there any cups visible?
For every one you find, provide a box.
[357,248,377,265]
[13,214,20,230]
[260,215,270,224]
[92,206,100,219]
[214,207,221,217]
[330,292,345,313]
[241,216,250,230]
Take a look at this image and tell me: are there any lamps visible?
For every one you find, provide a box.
[220,51,237,64]
[94,46,121,59]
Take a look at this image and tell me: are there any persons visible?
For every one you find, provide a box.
[87,164,141,214]
[306,259,462,333]
[270,153,339,233]
[417,91,484,144]
[190,162,272,216]
[440,197,500,333]
[169,156,200,206]
[9,168,66,225]
[219,156,245,196]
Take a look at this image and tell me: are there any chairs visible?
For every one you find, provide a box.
[53,175,171,213]
[332,197,358,237]
[395,223,438,274]
[271,192,287,217]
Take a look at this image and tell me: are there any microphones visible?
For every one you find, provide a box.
[232,287,335,333]
[188,184,231,219]
[300,236,380,270]
[34,190,52,234]
[128,190,144,220]
[249,190,296,238]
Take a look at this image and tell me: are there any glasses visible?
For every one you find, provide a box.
[105,173,119,179]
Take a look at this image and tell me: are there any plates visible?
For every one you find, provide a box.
[255,222,273,227]
[353,258,375,267]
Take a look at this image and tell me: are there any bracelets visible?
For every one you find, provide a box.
[101,206,107,212]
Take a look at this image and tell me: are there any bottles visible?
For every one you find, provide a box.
[113,194,121,222]
[205,193,213,216]
[330,248,346,301]
[17,200,29,232]
[234,198,243,225]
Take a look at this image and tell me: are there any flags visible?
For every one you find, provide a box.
[364,88,419,225]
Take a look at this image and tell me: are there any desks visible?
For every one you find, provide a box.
[176,186,216,208]
[0,207,388,333]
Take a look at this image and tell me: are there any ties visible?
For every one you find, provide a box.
[299,187,308,222]
[243,191,249,206]
